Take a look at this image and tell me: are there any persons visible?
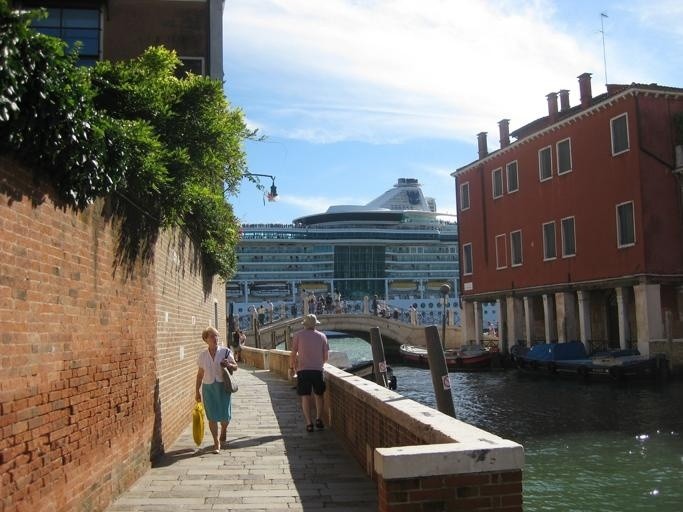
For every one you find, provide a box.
[195,327,238,454]
[289,314,329,432]
[233,289,416,362]
[488,321,494,336]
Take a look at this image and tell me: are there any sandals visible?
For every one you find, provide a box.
[306,424,314,432]
[316,419,324,428]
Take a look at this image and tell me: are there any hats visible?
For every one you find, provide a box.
[301,314,321,326]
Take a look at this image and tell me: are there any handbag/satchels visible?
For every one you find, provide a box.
[220,362,238,393]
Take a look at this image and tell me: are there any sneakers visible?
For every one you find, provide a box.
[213,440,226,454]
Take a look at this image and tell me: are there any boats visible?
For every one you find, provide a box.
[399,341,499,370]
[509,341,668,385]
[340,356,398,391]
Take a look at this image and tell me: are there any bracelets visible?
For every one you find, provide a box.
[289,368,294,369]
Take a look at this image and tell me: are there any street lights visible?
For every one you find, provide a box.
[284,279,301,303]
[598,7,612,86]
[414,280,428,298]
[438,284,451,348]
[235,280,254,304]
